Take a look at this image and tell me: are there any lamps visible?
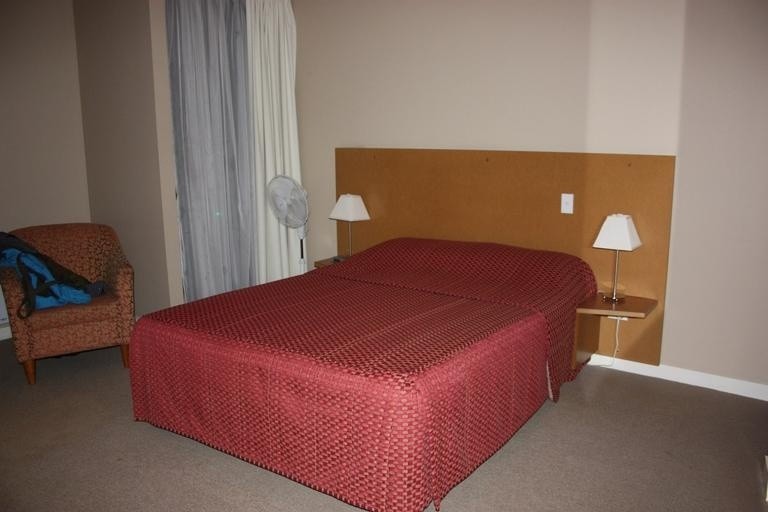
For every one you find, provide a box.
[590,212,644,306]
[327,191,371,263]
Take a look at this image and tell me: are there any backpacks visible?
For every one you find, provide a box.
[0,231,106,320]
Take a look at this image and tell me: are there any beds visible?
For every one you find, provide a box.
[127,146,678,512]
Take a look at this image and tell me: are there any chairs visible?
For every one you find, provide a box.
[0,223,134,384]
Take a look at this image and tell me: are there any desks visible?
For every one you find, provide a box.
[314,257,346,268]
[570,292,659,369]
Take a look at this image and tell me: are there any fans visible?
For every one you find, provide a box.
[267,175,310,277]
